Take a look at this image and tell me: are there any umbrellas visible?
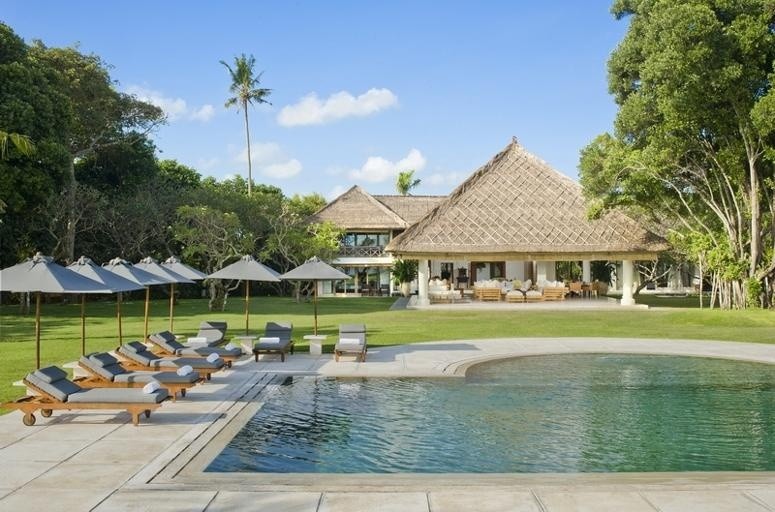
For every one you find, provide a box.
[0,250,206,369]
[206,255,281,335]
[278,255,352,336]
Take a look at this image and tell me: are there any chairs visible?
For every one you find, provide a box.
[428,279,599,304]
[253,320,296,365]
[334,323,368,363]
[1,321,241,428]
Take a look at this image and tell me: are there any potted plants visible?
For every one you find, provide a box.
[590,262,617,295]
[386,258,418,298]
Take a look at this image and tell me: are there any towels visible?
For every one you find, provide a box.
[259,337,280,344]
[339,338,360,346]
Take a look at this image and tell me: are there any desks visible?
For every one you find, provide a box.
[304,335,329,356]
[235,334,259,355]
